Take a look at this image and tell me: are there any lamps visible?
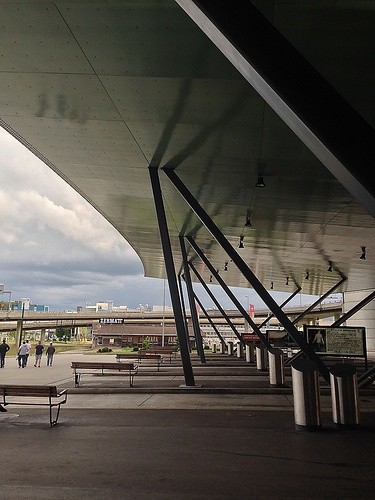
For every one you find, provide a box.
[244,216,253,228]
[359,246,366,260]
[285,277,289,285]
[270,282,273,289]
[238,236,244,248]
[223,262,229,271]
[209,275,212,282]
[305,271,309,279]
[256,177,265,187]
[326,261,333,272]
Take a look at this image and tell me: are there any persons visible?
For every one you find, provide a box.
[34,341,44,367]
[0,340,10,368]
[46,343,55,366]
[18,342,28,368]
[25,340,31,362]
[312,330,324,352]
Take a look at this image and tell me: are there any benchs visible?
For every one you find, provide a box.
[0,384,69,427]
[70,361,138,388]
[114,354,163,372]
[139,346,177,364]
